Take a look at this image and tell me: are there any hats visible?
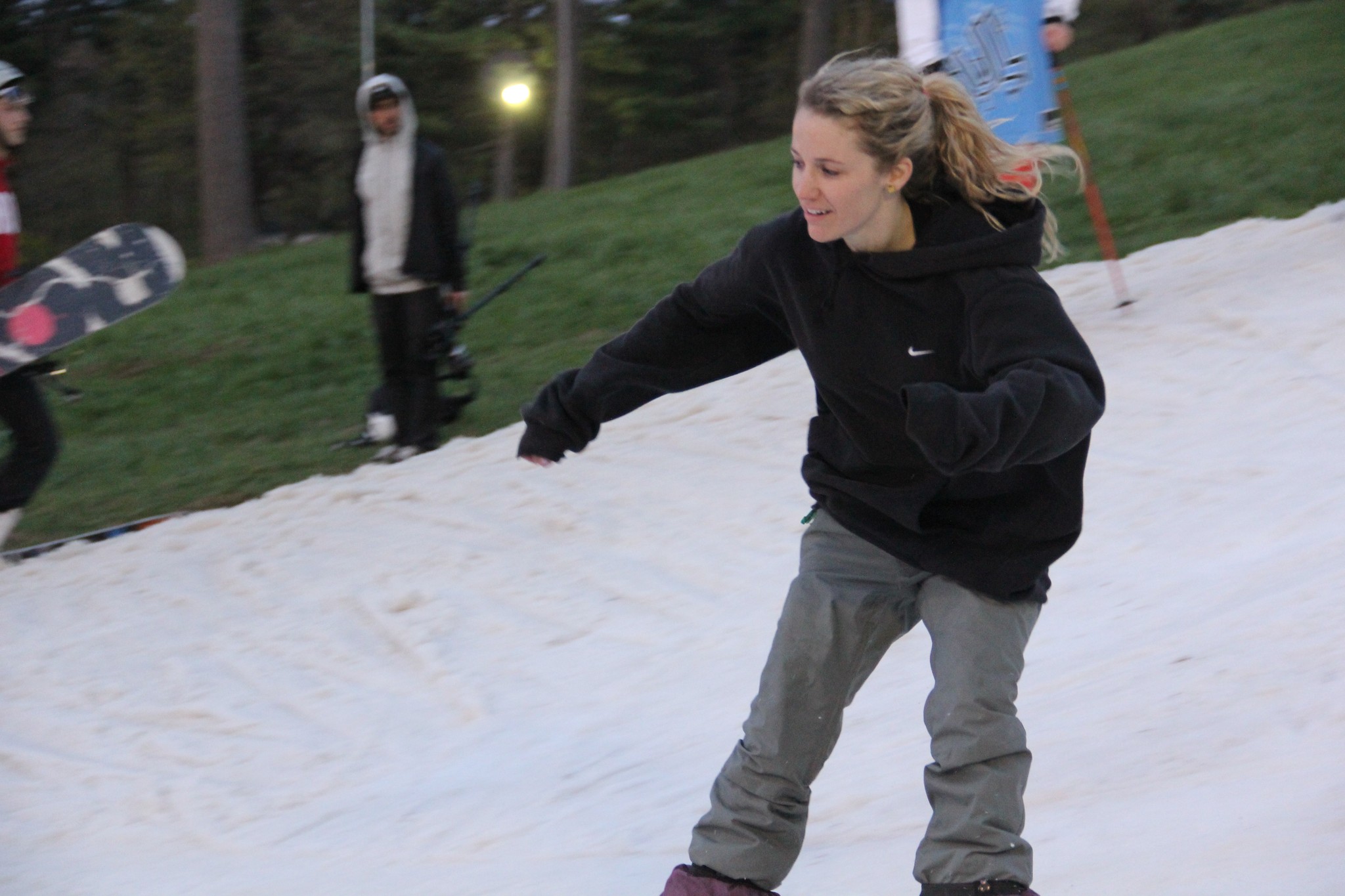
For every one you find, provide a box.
[366,85,399,100]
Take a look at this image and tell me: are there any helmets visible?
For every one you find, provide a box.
[0,60,27,99]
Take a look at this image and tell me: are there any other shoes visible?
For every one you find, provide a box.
[371,444,427,465]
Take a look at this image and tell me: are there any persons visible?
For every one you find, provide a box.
[0,58,65,553]
[889,0,1078,192]
[514,46,1106,896]
[344,75,472,465]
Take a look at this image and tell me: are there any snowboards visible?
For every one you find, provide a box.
[0,224,188,378]
